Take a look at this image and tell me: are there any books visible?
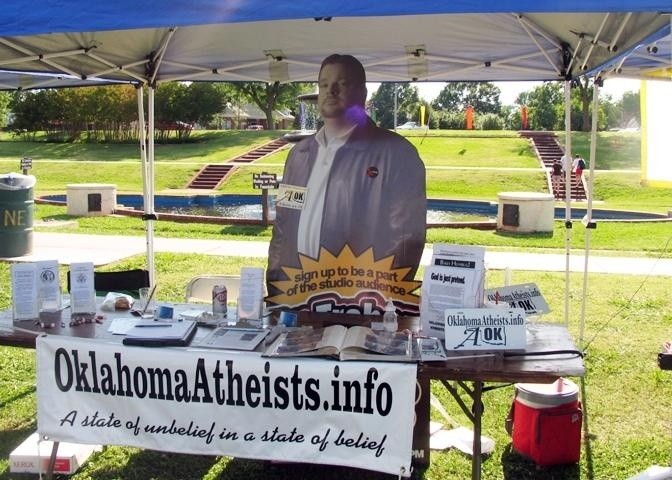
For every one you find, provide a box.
[123,320,198,347]
[260,324,422,363]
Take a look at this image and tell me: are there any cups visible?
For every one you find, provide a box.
[139,287,155,319]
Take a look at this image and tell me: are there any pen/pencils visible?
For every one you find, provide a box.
[134,324,172,327]
[141,283,159,315]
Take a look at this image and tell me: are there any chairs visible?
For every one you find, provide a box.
[66,269,269,308]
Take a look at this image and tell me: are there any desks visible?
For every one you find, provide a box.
[1,295,585,480]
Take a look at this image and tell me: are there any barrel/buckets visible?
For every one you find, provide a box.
[0,174,36,258]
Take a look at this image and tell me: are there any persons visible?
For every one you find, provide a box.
[551,153,586,198]
[266,54,426,309]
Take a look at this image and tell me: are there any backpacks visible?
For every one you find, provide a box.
[577,158,585,170]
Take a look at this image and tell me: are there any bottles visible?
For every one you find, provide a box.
[383,296,397,332]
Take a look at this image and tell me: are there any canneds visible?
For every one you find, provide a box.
[212,285,227,313]
